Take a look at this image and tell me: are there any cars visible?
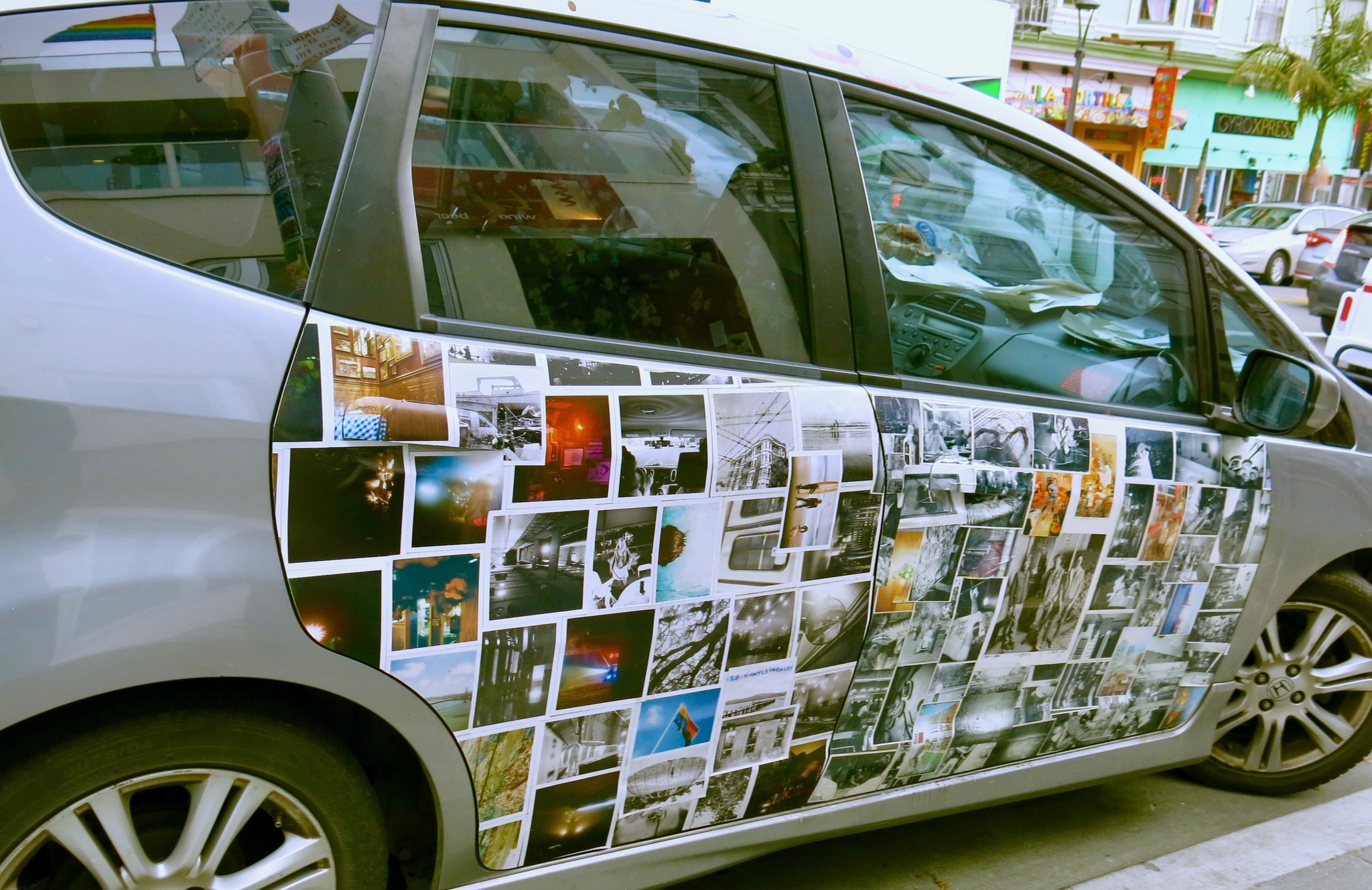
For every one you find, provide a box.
[1307,217,1372,336]
[1201,201,1365,284]
[1293,209,1372,284]
[1325,261,1372,390]
[669,441,696,448]
[559,79,1084,288]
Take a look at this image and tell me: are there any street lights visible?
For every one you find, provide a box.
[1063,0,1101,135]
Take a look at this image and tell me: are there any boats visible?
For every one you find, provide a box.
[1,0,1367,890]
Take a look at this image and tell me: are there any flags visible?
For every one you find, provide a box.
[43,13,157,42]
[674,703,698,747]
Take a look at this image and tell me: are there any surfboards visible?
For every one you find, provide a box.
[796,499,830,509]
[788,526,803,540]
[798,482,839,494]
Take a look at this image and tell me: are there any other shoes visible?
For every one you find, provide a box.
[1001,635,1058,650]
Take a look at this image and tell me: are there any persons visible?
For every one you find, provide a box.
[1198,194,1206,225]
[716,417,1263,806]
[658,525,685,568]
[1162,191,1170,201]
[609,540,640,601]
